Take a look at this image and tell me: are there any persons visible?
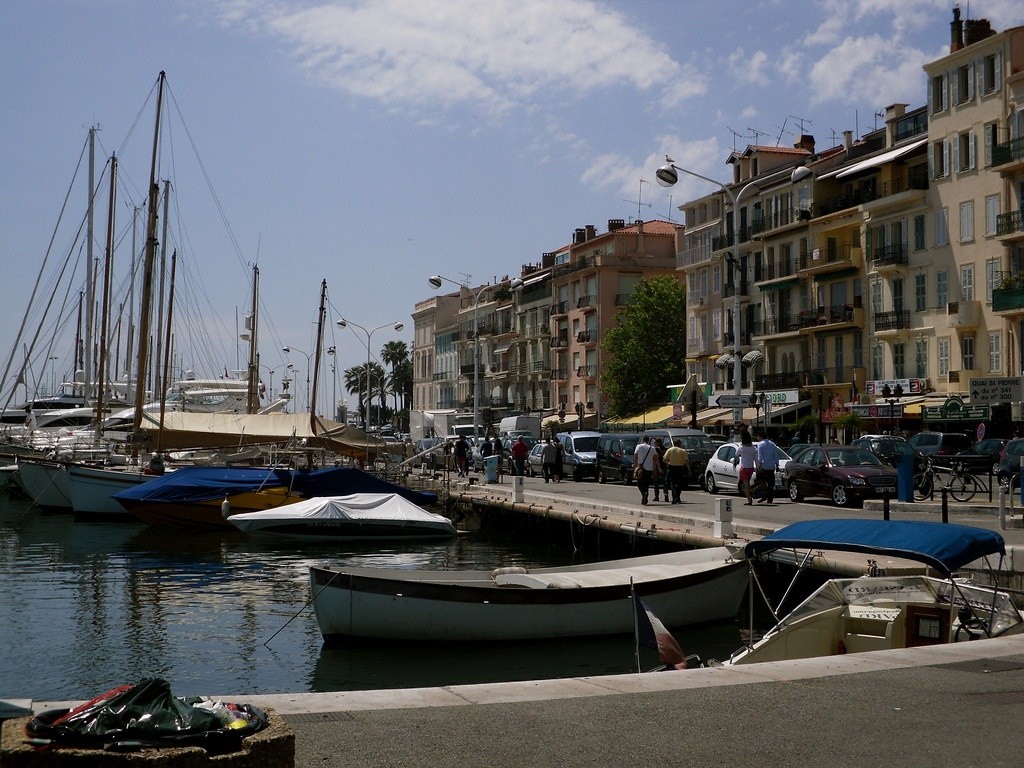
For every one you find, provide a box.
[727,423,747,443]
[652,437,670,502]
[492,433,503,465]
[634,435,659,505]
[511,435,528,476]
[480,436,492,472]
[791,431,800,445]
[541,437,564,483]
[663,438,688,504]
[453,434,472,477]
[394,436,417,467]
[830,436,839,444]
[734,431,780,506]
[806,434,813,444]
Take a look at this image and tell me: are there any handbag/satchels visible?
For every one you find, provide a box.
[633,466,644,479]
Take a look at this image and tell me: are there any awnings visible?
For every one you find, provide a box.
[814,138,929,181]
[494,343,513,355]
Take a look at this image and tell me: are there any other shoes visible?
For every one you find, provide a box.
[665,497,669,502]
[758,498,765,503]
[767,499,772,503]
[744,502,752,505]
[653,497,659,501]
[642,497,648,504]
[673,498,682,504]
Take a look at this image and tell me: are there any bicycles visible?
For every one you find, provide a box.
[913,453,978,502]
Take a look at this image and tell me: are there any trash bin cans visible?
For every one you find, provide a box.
[1,706,296,768]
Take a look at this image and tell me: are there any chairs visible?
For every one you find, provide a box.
[846,454,858,464]
[689,439,699,449]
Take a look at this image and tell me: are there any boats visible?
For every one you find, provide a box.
[226,492,473,541]
[631,517,1024,672]
[307,537,758,649]
[109,464,440,537]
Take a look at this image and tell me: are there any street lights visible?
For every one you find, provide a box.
[337,318,404,433]
[428,275,525,442]
[654,153,815,425]
[282,345,336,412]
[259,361,294,410]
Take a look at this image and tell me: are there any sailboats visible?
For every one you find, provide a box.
[0,70,411,517]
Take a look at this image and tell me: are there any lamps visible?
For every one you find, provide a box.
[721,252,741,272]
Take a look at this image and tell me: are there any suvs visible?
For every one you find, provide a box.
[850,434,933,493]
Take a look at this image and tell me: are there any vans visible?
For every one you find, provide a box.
[907,431,971,472]
[594,432,663,488]
[635,428,717,490]
[555,430,604,483]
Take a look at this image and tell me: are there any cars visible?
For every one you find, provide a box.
[786,443,840,461]
[707,433,732,450]
[950,438,1013,476]
[349,423,560,479]
[704,441,793,499]
[783,445,897,508]
[997,437,1024,494]
